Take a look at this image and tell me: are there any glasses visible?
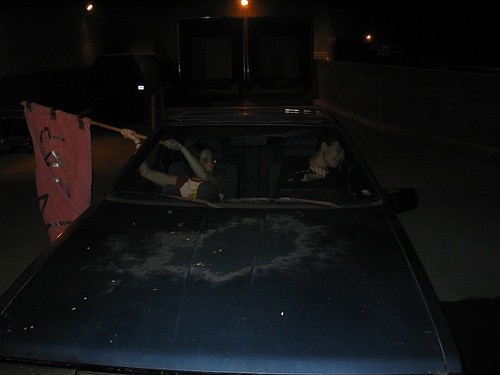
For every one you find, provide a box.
[200,159,217,167]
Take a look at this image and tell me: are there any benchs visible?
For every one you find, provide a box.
[228,135,273,197]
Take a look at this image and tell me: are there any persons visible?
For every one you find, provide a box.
[120,128,227,202]
[283,135,347,195]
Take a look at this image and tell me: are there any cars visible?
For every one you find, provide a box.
[1,107,464,374]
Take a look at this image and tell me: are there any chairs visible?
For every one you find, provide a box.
[268,140,325,196]
[161,138,236,200]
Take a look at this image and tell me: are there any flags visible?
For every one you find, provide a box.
[22,101,93,246]
